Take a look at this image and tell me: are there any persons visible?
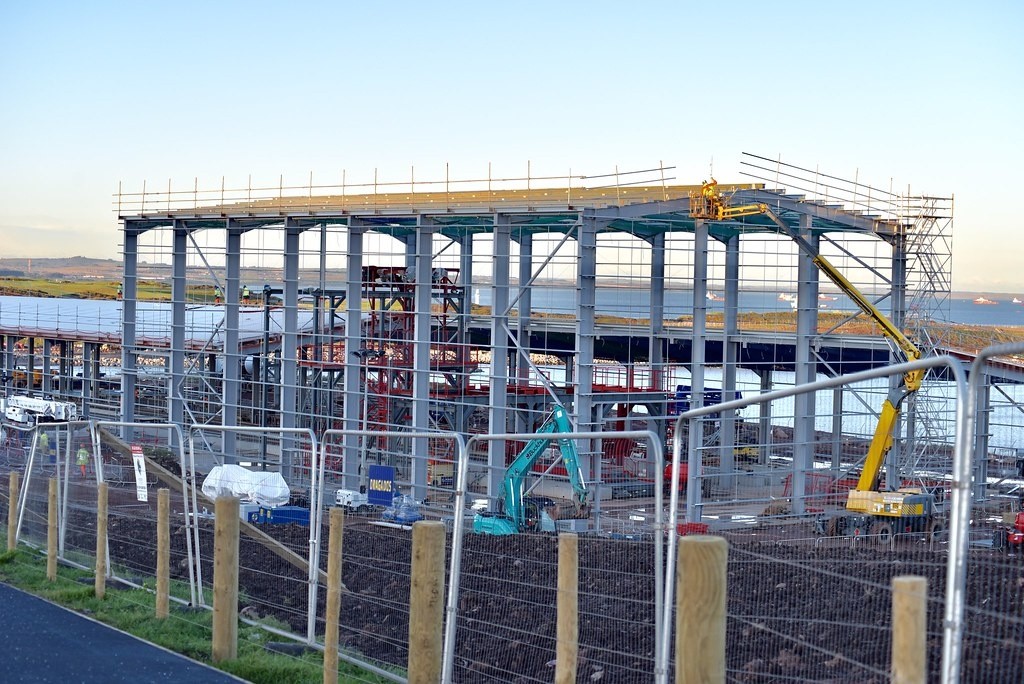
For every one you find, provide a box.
[243,285,250,304]
[214,285,220,304]
[118,284,122,298]
[76,444,89,478]
[40,430,50,462]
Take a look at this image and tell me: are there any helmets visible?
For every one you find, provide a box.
[80,444,86,448]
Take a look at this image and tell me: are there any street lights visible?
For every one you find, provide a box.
[349,347,386,494]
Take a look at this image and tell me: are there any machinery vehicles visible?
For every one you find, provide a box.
[0,395,91,456]
[472,404,592,536]
[689,189,947,547]
